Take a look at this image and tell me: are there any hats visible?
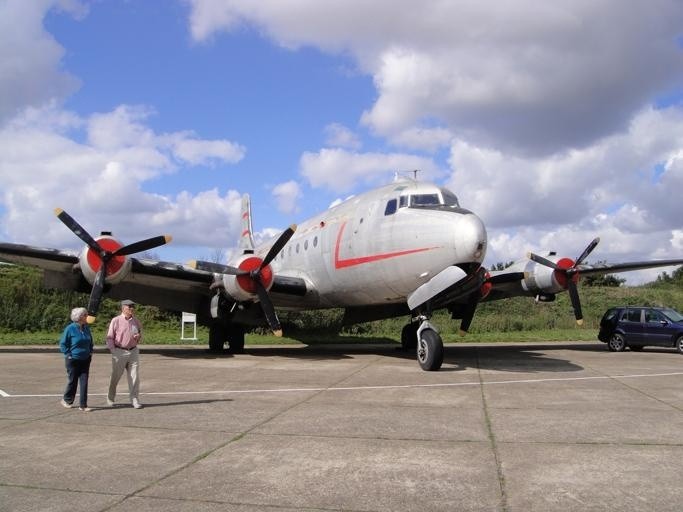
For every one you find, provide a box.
[120,299,136,306]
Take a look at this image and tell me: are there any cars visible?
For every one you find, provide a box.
[598,305,682,356]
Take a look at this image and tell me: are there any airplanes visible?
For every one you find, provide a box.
[0,170,683,371]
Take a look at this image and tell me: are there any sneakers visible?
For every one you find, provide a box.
[106,394,143,408]
[61,398,92,411]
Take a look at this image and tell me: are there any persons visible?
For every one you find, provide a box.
[59,307,94,411]
[106,300,144,409]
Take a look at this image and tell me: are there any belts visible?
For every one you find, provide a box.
[114,343,135,350]
